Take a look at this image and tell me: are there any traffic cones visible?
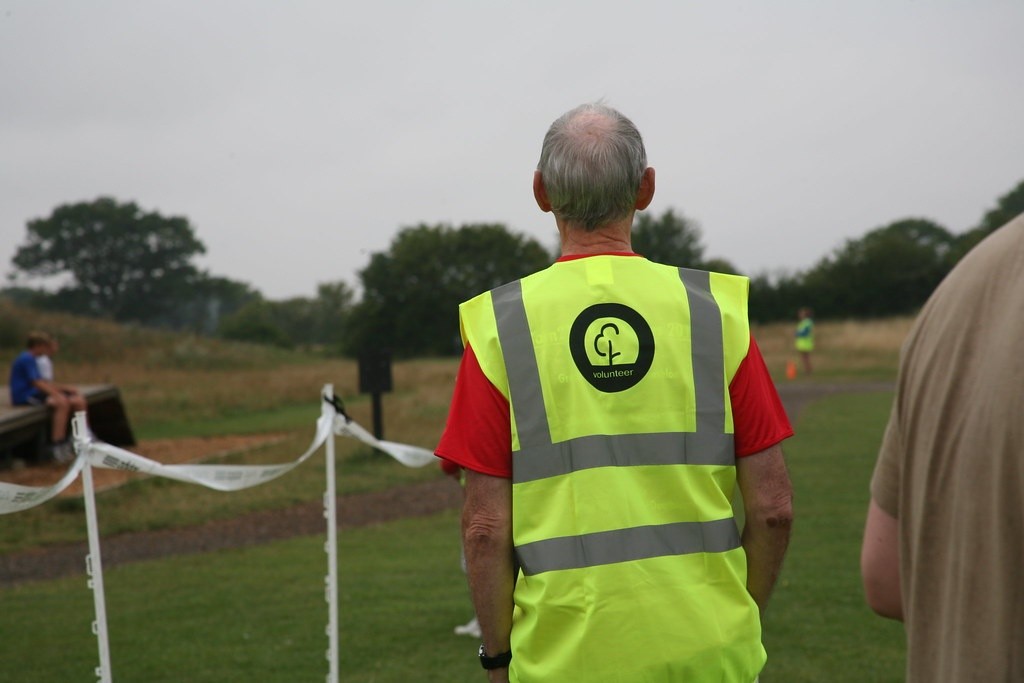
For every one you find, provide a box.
[784,360,798,382]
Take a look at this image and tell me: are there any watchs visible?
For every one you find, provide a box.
[479,644,512,670]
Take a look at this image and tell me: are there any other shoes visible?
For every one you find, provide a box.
[454,618,483,638]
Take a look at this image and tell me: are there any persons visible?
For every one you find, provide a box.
[10,331,87,463]
[434,104,794,683]
[794,309,813,373]
[861,213,1024,683]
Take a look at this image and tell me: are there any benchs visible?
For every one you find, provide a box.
[0,382,138,474]
